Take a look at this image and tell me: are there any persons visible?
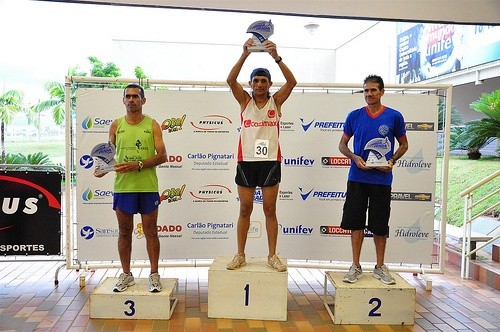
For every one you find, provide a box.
[94,84,168,293]
[339,76,409,285]
[227,38,288,273]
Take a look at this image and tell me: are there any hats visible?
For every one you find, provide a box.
[250,68,271,81]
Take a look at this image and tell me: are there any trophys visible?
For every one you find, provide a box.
[364,137,391,168]
[247,19,274,52]
[91,141,118,174]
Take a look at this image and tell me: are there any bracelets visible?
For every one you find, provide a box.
[275,56,282,63]
[391,157,395,164]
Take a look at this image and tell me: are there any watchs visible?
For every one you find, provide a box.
[137,160,143,171]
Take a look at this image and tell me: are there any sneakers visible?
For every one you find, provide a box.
[268,254,287,272]
[342,265,363,282]
[372,264,396,284]
[113,272,135,292]
[149,273,163,291]
[226,254,246,269]
